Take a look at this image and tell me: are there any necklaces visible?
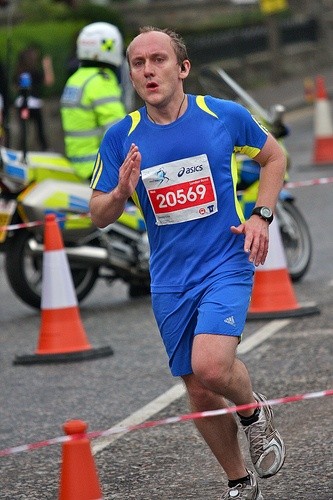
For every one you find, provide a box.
[147,95,186,123]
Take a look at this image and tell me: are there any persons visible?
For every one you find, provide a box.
[60,22,152,301]
[0,42,55,156]
[90,28,288,500]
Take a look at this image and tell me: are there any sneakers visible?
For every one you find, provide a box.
[220,470,264,500]
[237,391,287,479]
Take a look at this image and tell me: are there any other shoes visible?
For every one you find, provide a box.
[127,284,151,298]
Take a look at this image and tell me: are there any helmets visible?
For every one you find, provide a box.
[77,21,125,68]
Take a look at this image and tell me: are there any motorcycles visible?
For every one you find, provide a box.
[1,63,315,314]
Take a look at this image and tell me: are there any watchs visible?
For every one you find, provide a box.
[252,206,273,224]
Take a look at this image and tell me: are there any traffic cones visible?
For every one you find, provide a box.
[10,210,114,366]
[243,206,322,322]
[55,417,105,500]
[298,73,333,173]
